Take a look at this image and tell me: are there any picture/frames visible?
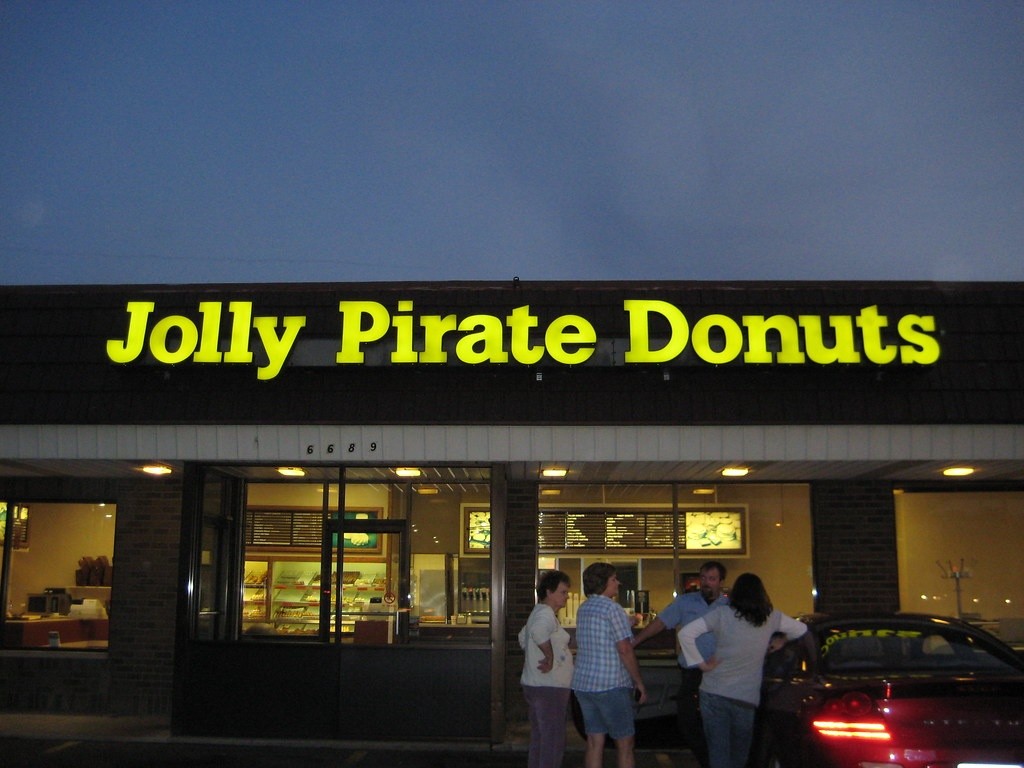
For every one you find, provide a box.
[682,573,701,594]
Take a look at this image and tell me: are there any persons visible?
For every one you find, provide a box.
[677,573,807,768]
[518,570,576,768]
[570,562,649,768]
[633,561,730,768]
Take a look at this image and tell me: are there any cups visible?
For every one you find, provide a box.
[450,616,457,625]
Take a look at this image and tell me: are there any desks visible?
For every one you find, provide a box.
[5,618,110,648]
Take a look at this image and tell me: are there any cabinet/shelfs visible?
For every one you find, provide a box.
[244,569,387,638]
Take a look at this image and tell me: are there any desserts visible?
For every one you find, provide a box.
[351,533,369,546]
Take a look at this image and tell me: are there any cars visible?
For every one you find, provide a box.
[755,611,1024,768]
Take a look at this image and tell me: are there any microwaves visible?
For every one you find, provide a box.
[26,592,71,616]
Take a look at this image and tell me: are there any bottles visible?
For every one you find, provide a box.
[461,584,490,611]
[48,631,60,647]
[458,613,471,624]
